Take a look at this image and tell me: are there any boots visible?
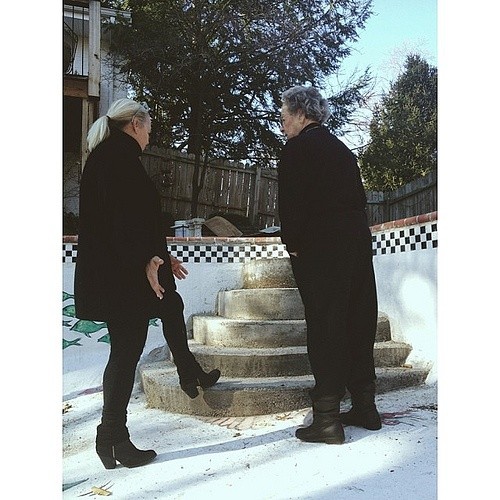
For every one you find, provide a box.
[96,407,157,470]
[173,351,221,399]
[295,391,345,444]
[338,383,382,431]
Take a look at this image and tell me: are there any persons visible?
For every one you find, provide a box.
[276,87,383,444]
[73,98,222,471]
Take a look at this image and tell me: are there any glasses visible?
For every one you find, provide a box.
[131,102,149,122]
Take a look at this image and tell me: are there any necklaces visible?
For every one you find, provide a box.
[305,126,321,133]
